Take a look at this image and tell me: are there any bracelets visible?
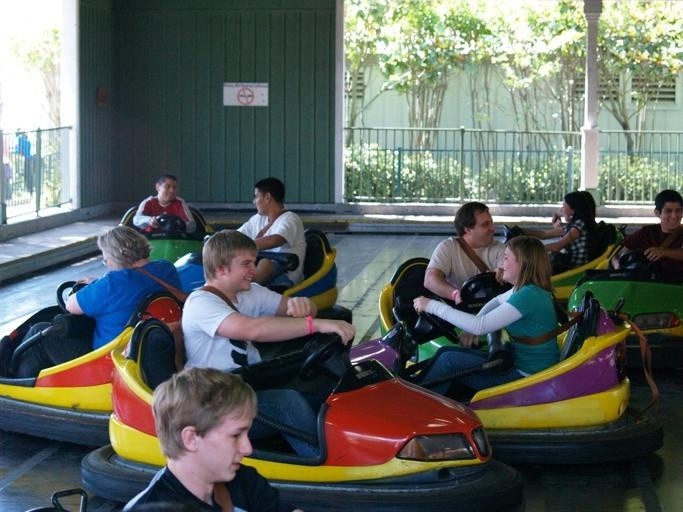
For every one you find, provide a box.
[450,289,459,302]
[305,315,314,335]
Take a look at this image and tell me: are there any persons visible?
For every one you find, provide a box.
[16,225,182,379]
[552,191,596,234]
[177,229,357,459]
[410,236,561,398]
[120,365,305,512]
[132,173,196,236]
[423,200,508,306]
[520,192,596,277]
[234,175,308,287]
[611,190,683,272]
